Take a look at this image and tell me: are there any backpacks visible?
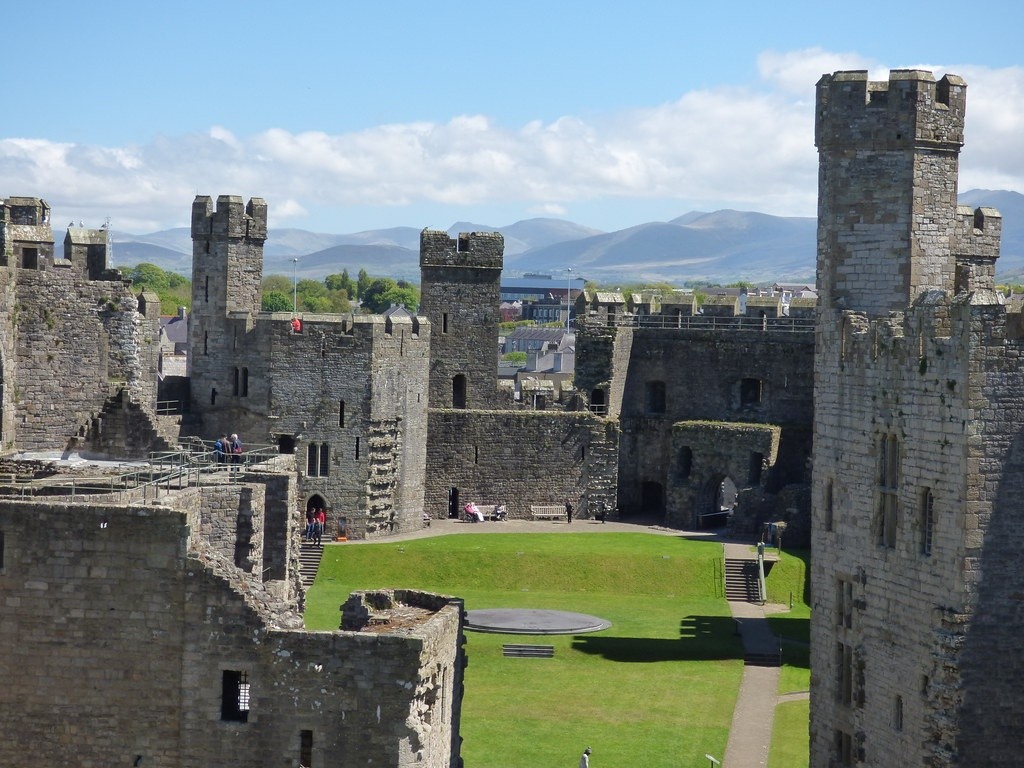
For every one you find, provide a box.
[214,439,224,458]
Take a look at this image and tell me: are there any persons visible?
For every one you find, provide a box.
[564,502,574,524]
[464,501,507,525]
[579,748,592,768]
[230,434,243,471]
[601,499,608,523]
[306,507,325,548]
[217,433,232,470]
[290,317,300,334]
[767,522,774,545]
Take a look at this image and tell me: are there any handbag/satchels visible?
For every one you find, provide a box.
[233,446,241,454]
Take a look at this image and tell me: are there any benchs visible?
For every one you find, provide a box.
[462,505,508,524]
[529,504,568,521]
